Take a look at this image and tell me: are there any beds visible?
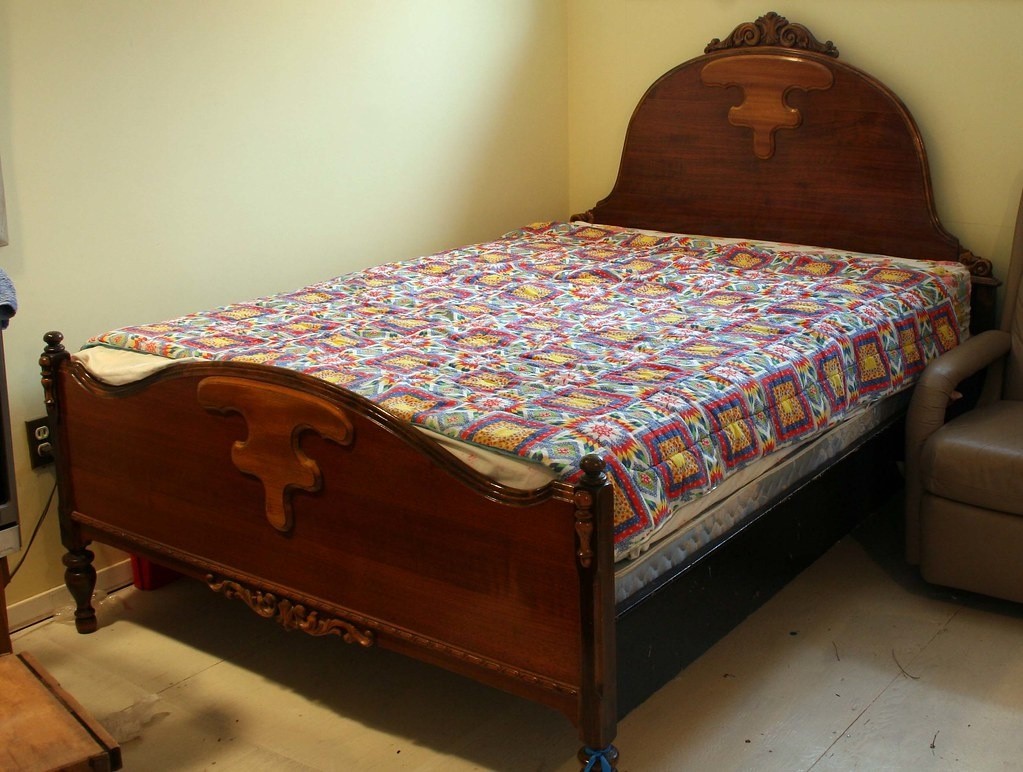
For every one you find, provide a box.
[39,10,1003,772]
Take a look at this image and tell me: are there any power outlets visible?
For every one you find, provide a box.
[25,414,58,470]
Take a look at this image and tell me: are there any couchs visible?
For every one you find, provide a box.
[903,187,1023,603]
